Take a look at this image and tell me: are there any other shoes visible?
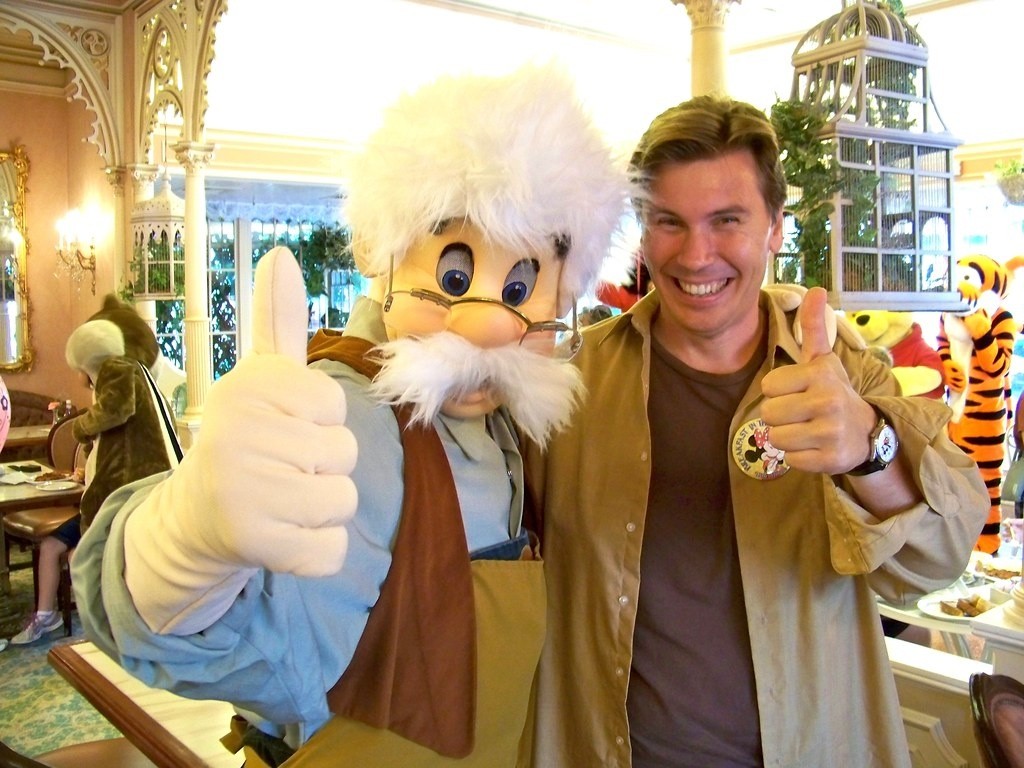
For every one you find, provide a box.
[0,639,9,651]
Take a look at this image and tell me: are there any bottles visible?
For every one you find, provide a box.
[62,399,74,417]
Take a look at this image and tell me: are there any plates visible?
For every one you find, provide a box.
[25,472,72,483]
[36,482,78,491]
[917,586,996,623]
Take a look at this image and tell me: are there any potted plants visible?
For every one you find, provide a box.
[771,0,921,291]
[303,223,356,296]
[993,157,1024,206]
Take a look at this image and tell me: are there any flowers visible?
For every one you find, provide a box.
[48,401,62,411]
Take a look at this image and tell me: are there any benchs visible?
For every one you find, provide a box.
[7,389,77,426]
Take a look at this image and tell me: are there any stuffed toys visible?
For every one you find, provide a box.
[844,311,948,438]
[937,253,1024,558]
[65,295,183,540]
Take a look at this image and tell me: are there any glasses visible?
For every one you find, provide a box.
[382,254,582,363]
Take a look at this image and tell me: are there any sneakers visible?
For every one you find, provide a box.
[10,611,64,644]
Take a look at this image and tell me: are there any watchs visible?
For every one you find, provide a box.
[847,417,899,476]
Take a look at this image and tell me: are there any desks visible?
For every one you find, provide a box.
[874,550,1024,660]
[47,639,243,768]
[0,459,86,595]
[4,423,57,460]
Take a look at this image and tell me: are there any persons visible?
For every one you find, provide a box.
[70,72,628,768]
[515,97,992,768]
[11,513,81,644]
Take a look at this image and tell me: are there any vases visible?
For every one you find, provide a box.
[52,408,59,427]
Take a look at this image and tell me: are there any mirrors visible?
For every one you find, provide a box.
[1,143,36,375]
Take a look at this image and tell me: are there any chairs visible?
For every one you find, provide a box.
[1,408,89,610]
[0,736,158,768]
